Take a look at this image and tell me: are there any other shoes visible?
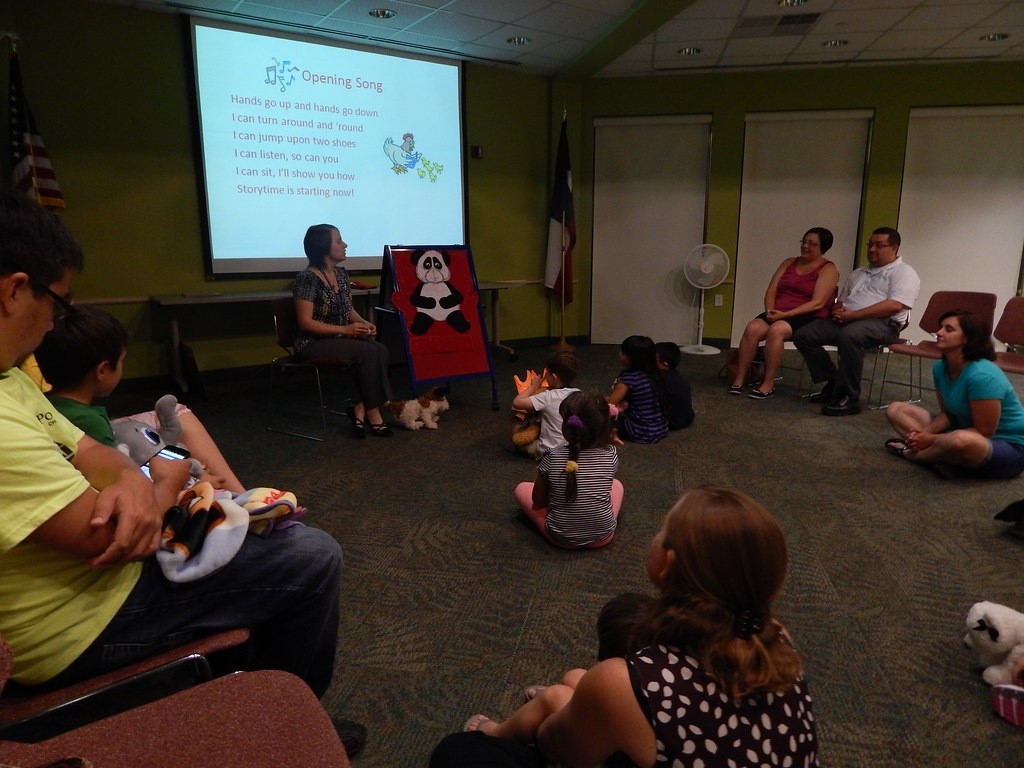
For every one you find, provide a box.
[332,717,368,758]
[728,384,744,394]
[748,386,776,398]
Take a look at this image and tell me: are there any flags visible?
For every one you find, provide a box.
[544,119,576,308]
[7,54,66,216]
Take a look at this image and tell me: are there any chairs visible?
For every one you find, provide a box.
[266,309,372,441]
[993,298,1024,376]
[878,292,997,409]
[0,638,351,766]
[742,272,840,397]
[0,626,251,730]
[806,290,912,409]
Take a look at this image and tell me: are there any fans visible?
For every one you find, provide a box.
[680,243,731,355]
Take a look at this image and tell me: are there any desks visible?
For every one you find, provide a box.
[149,280,514,392]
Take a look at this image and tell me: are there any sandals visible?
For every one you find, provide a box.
[885,438,914,461]
[463,714,494,733]
[527,685,545,701]
[368,420,393,436]
[353,416,367,440]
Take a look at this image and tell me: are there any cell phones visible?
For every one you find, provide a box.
[141,444,191,479]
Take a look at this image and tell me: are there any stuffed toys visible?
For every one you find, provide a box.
[512,368,550,445]
[110,394,199,492]
[962,599,1024,685]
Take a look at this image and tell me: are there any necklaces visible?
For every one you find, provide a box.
[319,267,333,287]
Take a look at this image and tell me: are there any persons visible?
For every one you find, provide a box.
[728,227,840,397]
[427,485,821,768]
[0,189,368,754]
[292,224,395,438]
[793,228,913,417]
[513,351,584,459]
[883,309,1024,480]
[605,334,669,444]
[515,388,624,549]
[654,341,695,432]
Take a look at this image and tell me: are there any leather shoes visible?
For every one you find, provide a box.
[810,392,826,404]
[823,396,861,415]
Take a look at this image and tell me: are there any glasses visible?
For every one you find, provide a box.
[41,285,78,328]
[867,241,891,249]
[800,239,821,248]
